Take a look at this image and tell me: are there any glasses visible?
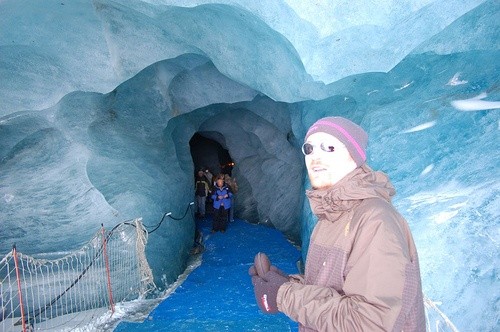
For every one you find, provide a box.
[302,142,336,155]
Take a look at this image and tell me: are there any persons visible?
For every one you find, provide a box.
[209,179,235,234]
[194,168,212,217]
[203,167,238,224]
[249,115,426,331]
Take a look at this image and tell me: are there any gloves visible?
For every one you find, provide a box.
[248,264,292,287]
[255,271,290,313]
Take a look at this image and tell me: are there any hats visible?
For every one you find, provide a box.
[304,116,368,168]
